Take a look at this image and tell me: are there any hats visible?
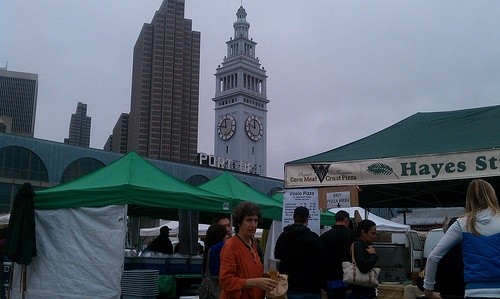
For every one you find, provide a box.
[294,206,312,219]
[160,226,172,232]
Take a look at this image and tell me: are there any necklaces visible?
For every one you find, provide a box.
[235,231,257,263]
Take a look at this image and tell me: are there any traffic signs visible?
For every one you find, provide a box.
[326,191,351,209]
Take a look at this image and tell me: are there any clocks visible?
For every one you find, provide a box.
[245,115,264,141]
[217,115,236,140]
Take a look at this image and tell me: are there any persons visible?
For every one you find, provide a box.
[320,210,354,299]
[219,202,278,299]
[274,206,322,299]
[202,217,232,276]
[147,226,173,254]
[417,217,465,299]
[423,179,500,299]
[349,220,378,299]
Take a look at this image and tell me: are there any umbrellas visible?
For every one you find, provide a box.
[5,182,37,299]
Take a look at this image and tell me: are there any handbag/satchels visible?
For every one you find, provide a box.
[198,248,219,299]
[342,242,381,288]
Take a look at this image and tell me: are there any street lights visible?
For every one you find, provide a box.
[396,209,413,225]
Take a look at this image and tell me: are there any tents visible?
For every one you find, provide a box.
[138,221,264,254]
[272,191,284,203]
[9,152,233,299]
[196,172,283,258]
[284,104,500,188]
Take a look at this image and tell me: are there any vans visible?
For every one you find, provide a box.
[421,227,444,272]
[370,228,423,285]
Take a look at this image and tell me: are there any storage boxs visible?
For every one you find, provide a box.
[318,186,362,208]
